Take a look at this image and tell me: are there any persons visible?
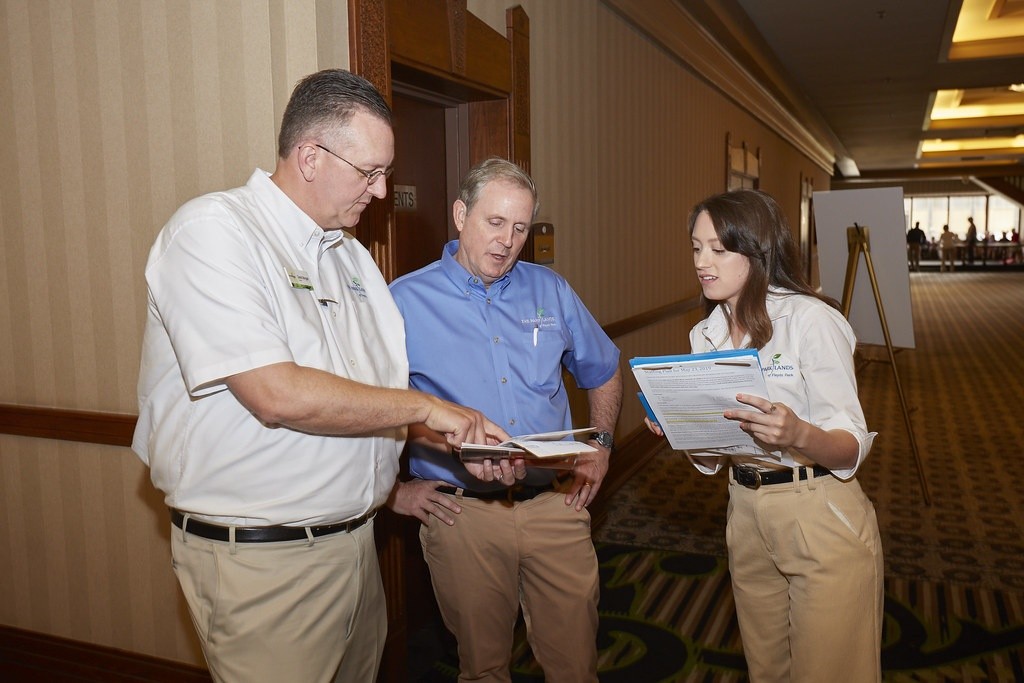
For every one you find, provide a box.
[643,188,886,683]
[388,155,624,683]
[907,216,1024,272]
[130,69,528,683]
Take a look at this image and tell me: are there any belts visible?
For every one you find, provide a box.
[435,475,568,501]
[733,463,830,490]
[171,507,377,542]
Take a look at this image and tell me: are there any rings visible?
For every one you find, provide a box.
[767,403,776,414]
[583,480,592,488]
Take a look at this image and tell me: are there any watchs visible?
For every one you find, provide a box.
[588,430,616,452]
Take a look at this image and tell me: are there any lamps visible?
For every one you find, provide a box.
[839,155,861,177]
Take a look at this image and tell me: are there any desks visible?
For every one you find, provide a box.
[906,242,1017,265]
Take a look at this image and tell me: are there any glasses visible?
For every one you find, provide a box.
[298,144,394,183]
